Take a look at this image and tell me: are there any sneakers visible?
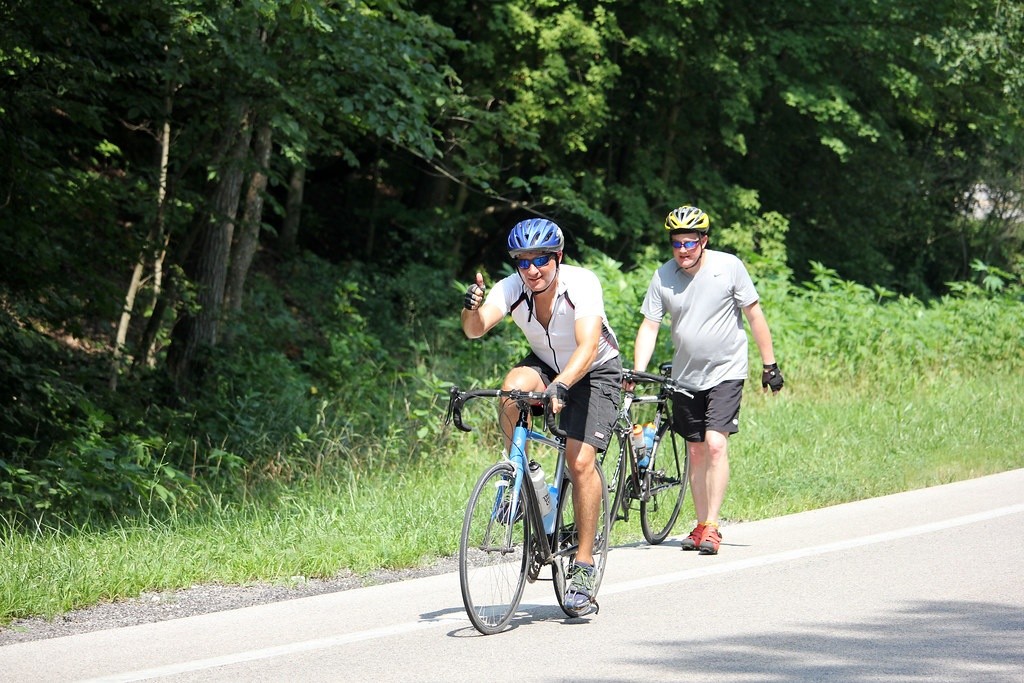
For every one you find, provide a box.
[681,524,705,550]
[564,556,597,608]
[699,525,721,555]
[497,486,520,523]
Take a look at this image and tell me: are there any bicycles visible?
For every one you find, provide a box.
[443,384,610,635]
[591,360,690,556]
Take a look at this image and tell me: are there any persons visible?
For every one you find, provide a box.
[621,204,784,556]
[460,218,621,619]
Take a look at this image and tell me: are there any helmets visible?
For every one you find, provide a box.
[665,205,709,233]
[508,218,564,252]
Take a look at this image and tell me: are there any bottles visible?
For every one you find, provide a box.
[637,421,657,468]
[541,486,559,534]
[633,424,646,459]
[529,459,552,517]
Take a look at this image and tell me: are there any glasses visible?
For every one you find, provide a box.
[515,253,555,269]
[671,240,700,248]
